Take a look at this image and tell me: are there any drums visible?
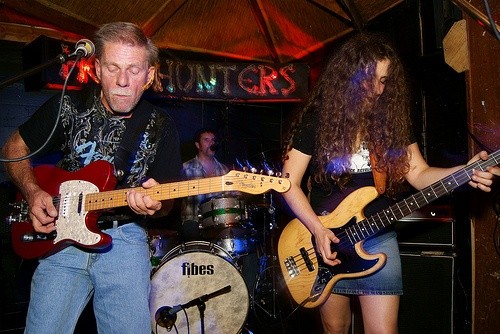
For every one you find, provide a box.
[197,195,246,236]
[147,240,252,333]
[204,238,251,257]
[241,207,278,239]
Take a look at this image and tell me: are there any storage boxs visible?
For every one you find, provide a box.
[400,249,458,334]
[396,218,456,248]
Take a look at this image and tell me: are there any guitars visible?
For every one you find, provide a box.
[10,161,291,257]
[274,151,500,313]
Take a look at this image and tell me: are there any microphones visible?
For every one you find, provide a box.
[159,309,171,322]
[210,139,228,151]
[74,38,96,56]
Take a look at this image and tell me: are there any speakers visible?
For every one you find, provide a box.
[347,251,457,334]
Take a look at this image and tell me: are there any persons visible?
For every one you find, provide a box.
[180,130,254,242]
[281,32,500,334]
[0,22,181,334]
